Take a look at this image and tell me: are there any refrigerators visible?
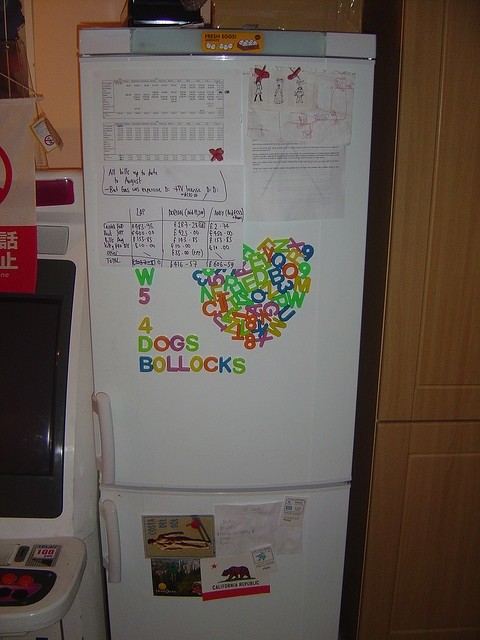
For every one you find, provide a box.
[78,20,376,634]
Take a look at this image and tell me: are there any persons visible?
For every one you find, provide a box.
[149,532,212,554]
